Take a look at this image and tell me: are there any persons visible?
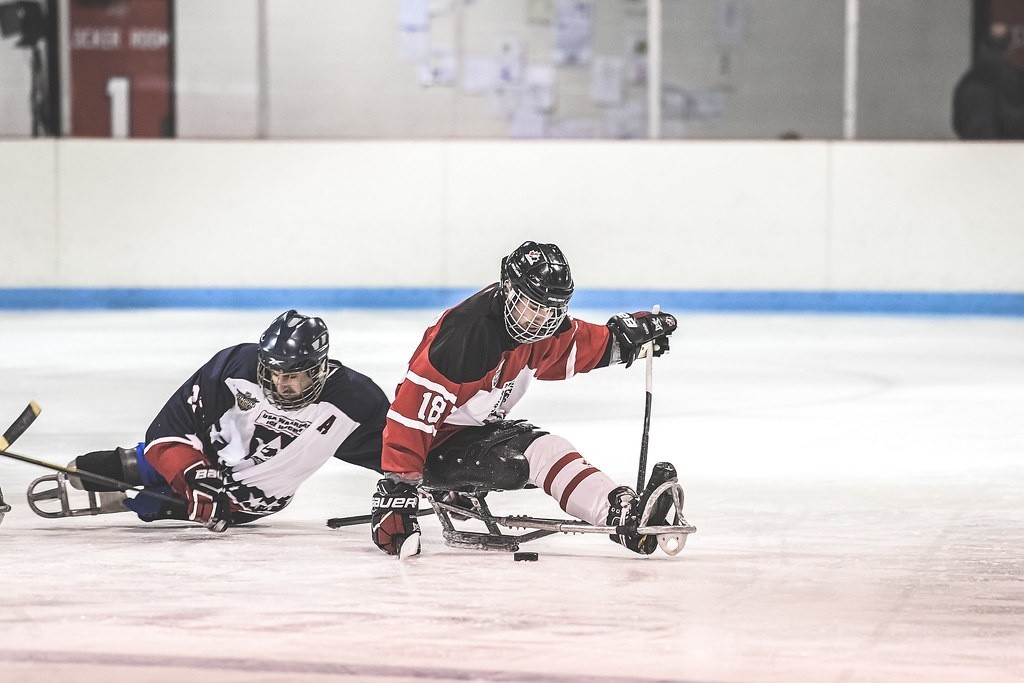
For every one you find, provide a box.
[64,310,391,532]
[370,241,677,559]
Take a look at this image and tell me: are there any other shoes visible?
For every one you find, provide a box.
[606,462,697,555]
[65,442,148,492]
[122,491,187,522]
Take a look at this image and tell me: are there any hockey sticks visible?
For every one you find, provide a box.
[0,400,188,510]
[325,508,435,529]
[635,304,660,495]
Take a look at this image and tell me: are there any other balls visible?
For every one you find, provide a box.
[513,552,539,562]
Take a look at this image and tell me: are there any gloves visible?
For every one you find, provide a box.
[606,311,677,368]
[371,471,423,557]
[145,442,230,531]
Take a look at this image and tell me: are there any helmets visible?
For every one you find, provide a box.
[501,241,574,312]
[258,310,329,373]
